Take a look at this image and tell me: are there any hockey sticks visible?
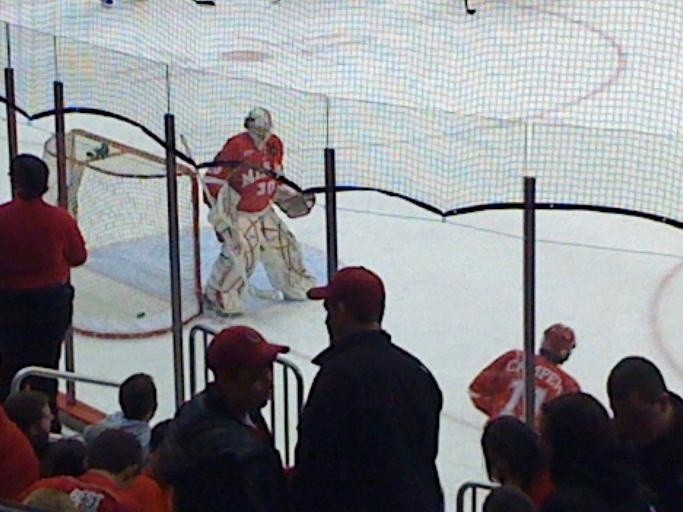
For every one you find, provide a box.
[179,136,285,301]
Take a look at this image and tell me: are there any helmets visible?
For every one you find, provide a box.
[540,324,575,359]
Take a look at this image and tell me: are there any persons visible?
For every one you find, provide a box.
[2,372,175,511]
[1,153,86,435]
[150,325,294,511]
[467,322,683,511]
[202,105,315,315]
[295,267,445,511]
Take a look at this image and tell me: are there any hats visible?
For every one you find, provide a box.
[205,326,289,371]
[307,266,384,313]
